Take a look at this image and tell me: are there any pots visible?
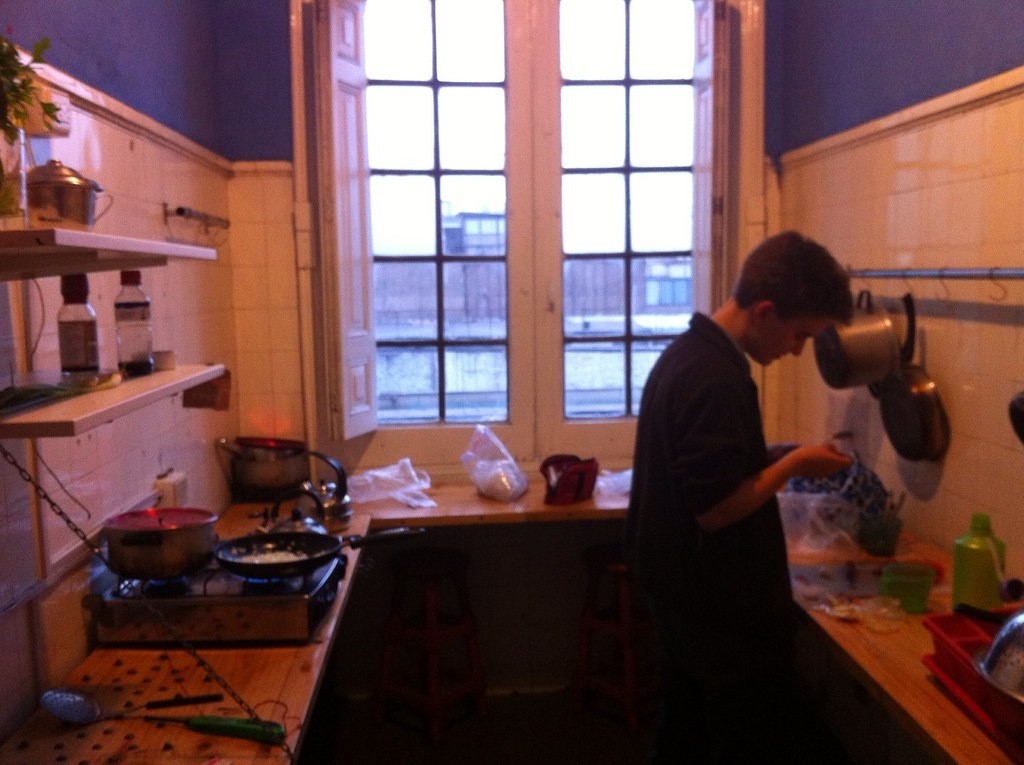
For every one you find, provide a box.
[212,524,430,580]
[97,507,220,582]
[26,157,102,228]
[814,289,953,461]
[219,436,310,500]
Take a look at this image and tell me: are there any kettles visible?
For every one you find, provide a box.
[290,449,354,534]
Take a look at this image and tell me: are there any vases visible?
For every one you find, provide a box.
[0,126,27,230]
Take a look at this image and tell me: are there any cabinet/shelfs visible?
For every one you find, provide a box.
[0,227,225,440]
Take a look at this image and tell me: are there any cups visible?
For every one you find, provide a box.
[856,516,902,555]
[876,563,937,616]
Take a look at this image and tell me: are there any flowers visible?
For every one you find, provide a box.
[0,26,64,139]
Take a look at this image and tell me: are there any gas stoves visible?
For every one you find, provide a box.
[88,550,348,643]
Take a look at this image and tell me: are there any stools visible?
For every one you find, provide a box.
[372,548,488,738]
[574,541,665,737]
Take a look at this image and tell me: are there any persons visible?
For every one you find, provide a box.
[627,232,853,765]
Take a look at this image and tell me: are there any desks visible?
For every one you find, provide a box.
[371,468,1024,765]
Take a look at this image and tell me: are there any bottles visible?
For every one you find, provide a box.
[953,510,1008,610]
[114,269,151,374]
[57,273,98,381]
